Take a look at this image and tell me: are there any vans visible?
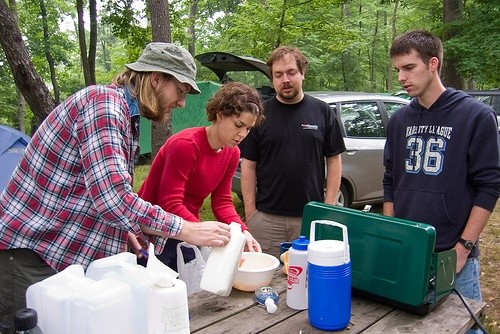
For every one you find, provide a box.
[194,50,413,209]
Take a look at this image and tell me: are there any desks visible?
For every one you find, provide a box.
[187,260,487,334]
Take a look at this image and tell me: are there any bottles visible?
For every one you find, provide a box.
[201,222,246,297]
[286,236,310,310]
[12,308,43,334]
[255,286,280,313]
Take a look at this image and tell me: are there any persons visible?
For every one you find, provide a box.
[237,46,347,260]
[0,41,201,334]
[383,29,500,334]
[128,82,262,280]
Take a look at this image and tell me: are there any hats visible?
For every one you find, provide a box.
[125,42,201,95]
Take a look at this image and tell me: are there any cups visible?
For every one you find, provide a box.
[280,242,292,264]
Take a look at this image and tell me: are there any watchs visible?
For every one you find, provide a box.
[457,237,476,250]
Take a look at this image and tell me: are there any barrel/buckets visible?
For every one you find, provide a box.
[307,220,352,331]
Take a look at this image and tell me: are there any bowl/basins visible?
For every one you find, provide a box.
[232,252,280,291]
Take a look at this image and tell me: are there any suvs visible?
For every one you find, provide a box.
[393,88,499,138]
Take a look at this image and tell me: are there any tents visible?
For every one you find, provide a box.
[0,124,31,195]
[139,81,223,166]
